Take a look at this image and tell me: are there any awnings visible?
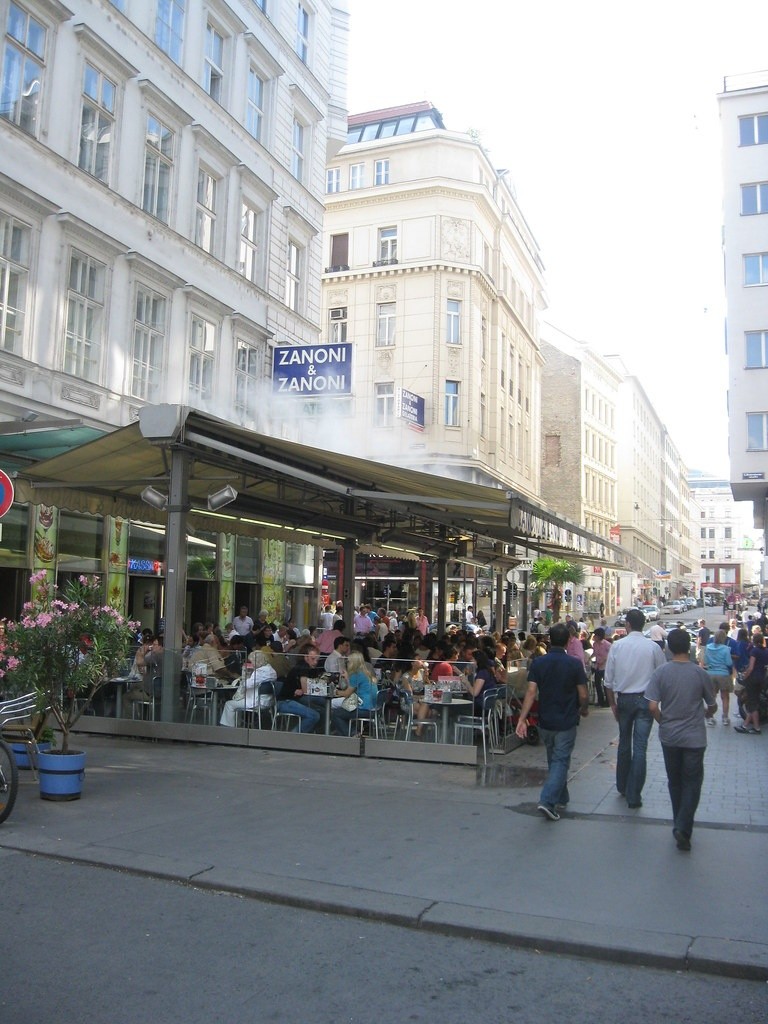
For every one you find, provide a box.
[0,405,657,721]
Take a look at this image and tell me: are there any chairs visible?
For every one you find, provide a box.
[73,674,509,766]
[0,688,52,745]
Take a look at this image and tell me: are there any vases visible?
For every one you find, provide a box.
[11,742,50,772]
[36,750,86,802]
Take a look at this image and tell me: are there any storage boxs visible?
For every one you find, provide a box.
[206,677,217,688]
[433,690,443,702]
[306,678,312,694]
[311,678,328,696]
[442,692,452,703]
[191,664,207,686]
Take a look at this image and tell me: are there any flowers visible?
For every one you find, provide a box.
[0,568,144,753]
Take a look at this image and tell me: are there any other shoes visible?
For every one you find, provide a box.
[627,802,643,809]
[537,801,561,821]
[594,703,601,706]
[706,718,716,725]
[672,829,691,850]
[601,703,610,707]
[734,725,747,733]
[413,731,422,740]
[722,718,730,725]
[748,728,761,734]
[558,801,567,808]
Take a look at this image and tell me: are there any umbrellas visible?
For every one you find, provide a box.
[702,587,724,594]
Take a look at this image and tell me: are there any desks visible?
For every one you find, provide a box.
[190,684,238,727]
[419,698,474,744]
[303,693,340,736]
[102,677,142,718]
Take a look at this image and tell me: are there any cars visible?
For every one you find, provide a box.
[619,607,651,627]
[685,597,712,610]
[663,600,683,614]
[680,600,688,612]
[643,604,660,621]
[663,628,698,662]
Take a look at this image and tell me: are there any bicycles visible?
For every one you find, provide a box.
[0,737,19,825]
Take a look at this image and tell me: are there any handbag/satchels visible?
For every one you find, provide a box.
[342,693,364,712]
[232,676,249,701]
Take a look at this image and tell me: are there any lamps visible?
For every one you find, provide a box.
[15,411,39,422]
[634,503,640,511]
[679,534,683,537]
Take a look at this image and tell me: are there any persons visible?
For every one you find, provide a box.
[37,606,613,733]
[601,582,768,734]
[515,623,589,822]
[644,629,717,852]
[602,610,668,809]
